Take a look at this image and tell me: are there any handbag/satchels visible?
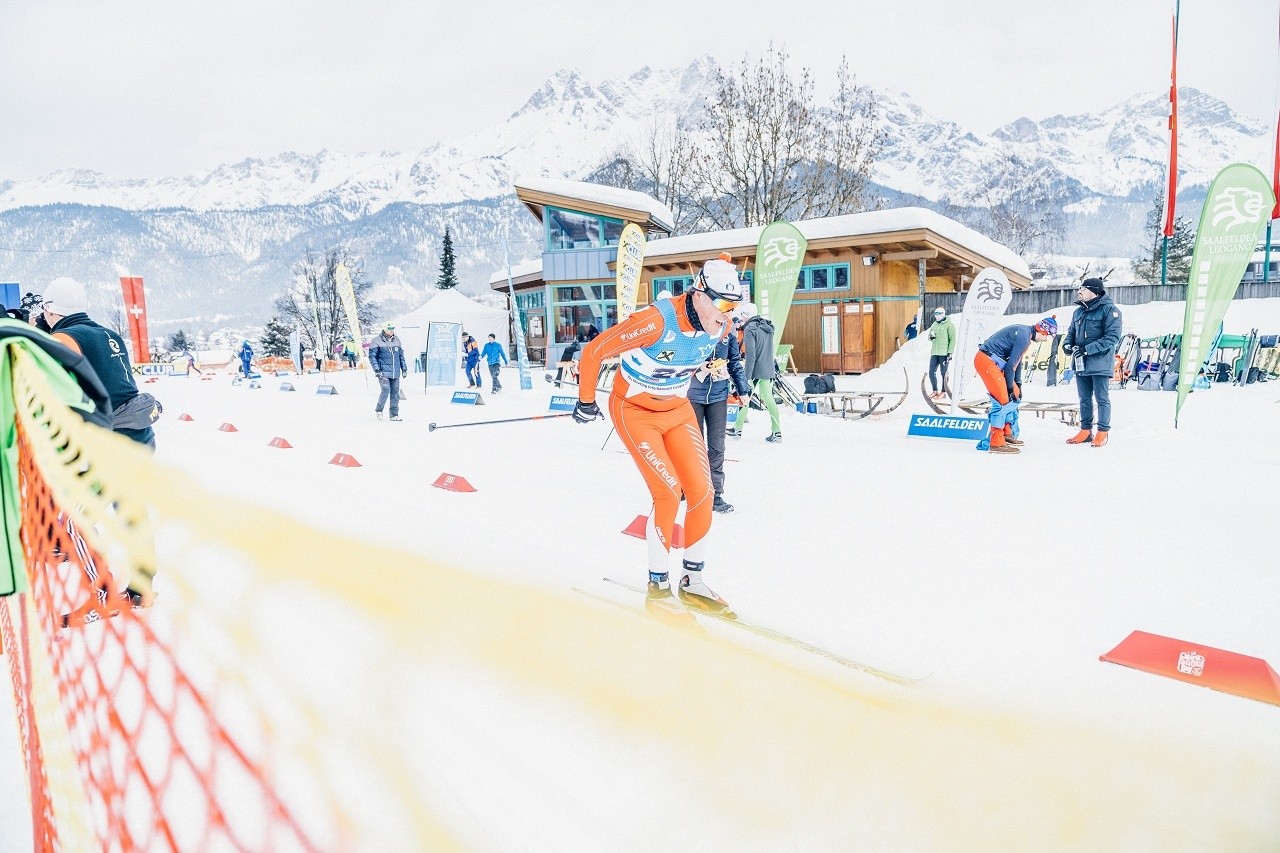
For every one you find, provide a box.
[108,394,164,428]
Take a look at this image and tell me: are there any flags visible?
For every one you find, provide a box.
[754,220,808,362]
[1159,11,1178,238]
[335,263,365,370]
[615,222,645,325]
[1269,10,1279,219]
[119,277,153,364]
[501,231,533,389]
[954,266,1014,401]
[1175,163,1277,429]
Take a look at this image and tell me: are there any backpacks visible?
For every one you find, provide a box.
[1106,356,1130,389]
[1114,334,1142,379]
[804,374,835,394]
[1137,344,1270,392]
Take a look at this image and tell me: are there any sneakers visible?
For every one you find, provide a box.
[1006,436,1024,446]
[928,391,939,398]
[937,392,945,399]
[468,384,474,388]
[1066,429,1093,443]
[475,385,481,389]
[647,571,691,618]
[1090,430,1108,446]
[988,445,1019,454]
[389,416,402,420]
[492,386,502,394]
[375,412,382,419]
[679,566,726,612]
[725,428,741,438]
[765,432,782,442]
[1079,286,1099,290]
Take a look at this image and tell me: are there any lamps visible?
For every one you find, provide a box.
[862,256,877,265]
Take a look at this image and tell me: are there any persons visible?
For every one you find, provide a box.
[554,325,600,388]
[571,259,742,623]
[313,341,322,370]
[186,352,203,377]
[478,333,507,394]
[238,341,254,378]
[657,289,674,301]
[462,332,482,390]
[300,342,305,370]
[681,332,750,513]
[344,335,359,370]
[42,276,157,629]
[369,321,407,421]
[927,305,956,400]
[726,302,783,443]
[1062,277,1122,447]
[974,315,1058,454]
[903,315,919,342]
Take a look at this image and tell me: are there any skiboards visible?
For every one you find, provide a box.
[571,572,937,712]
[1120,324,1261,392]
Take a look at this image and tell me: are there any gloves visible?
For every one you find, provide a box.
[35,313,52,334]
[1063,344,1073,357]
[1071,346,1086,358]
[570,402,604,423]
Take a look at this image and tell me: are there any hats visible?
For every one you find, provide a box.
[20,293,44,319]
[488,334,495,339]
[741,303,757,318]
[462,332,468,335]
[695,259,743,300]
[1083,277,1104,293]
[46,279,87,316]
[935,308,945,316]
[732,317,739,323]
[1035,314,1059,335]
[384,321,394,329]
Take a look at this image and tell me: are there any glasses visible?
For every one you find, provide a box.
[934,311,943,316]
[704,285,739,314]
[386,326,394,330]
[734,320,743,324]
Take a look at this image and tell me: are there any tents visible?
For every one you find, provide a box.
[378,287,511,370]
